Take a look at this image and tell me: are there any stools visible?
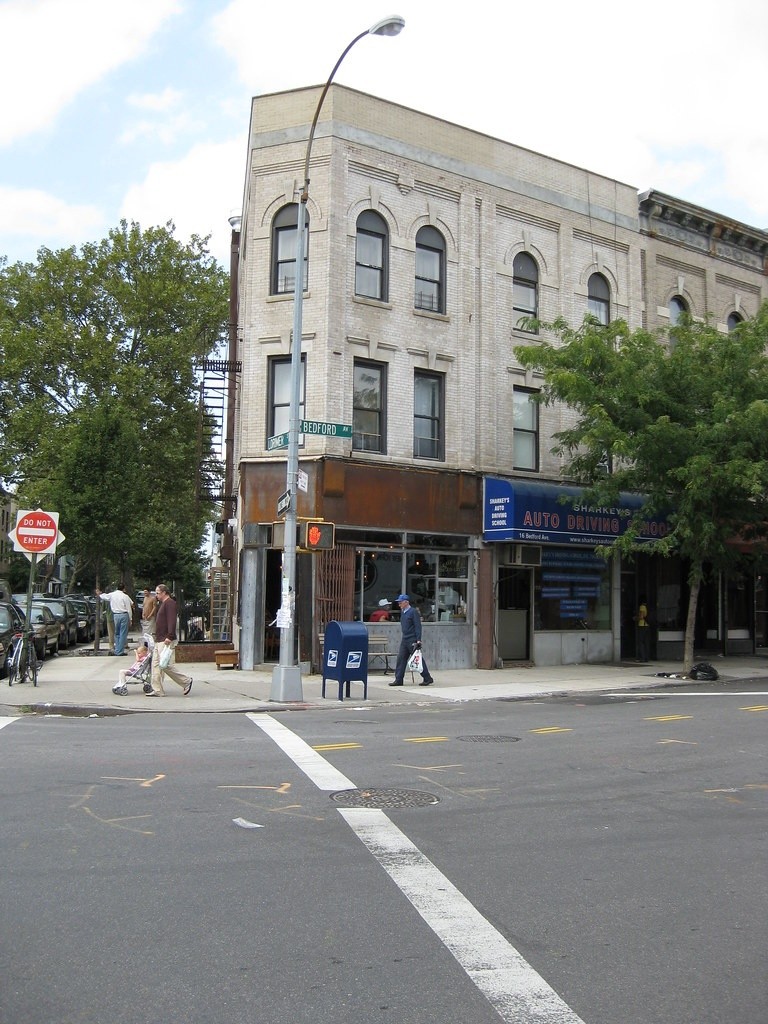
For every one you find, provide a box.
[214,650,239,669]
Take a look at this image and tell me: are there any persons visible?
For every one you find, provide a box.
[415,604,427,621]
[370,599,392,622]
[96,583,138,656]
[388,595,433,686]
[143,587,157,642]
[632,595,649,662]
[113,646,149,689]
[146,584,193,697]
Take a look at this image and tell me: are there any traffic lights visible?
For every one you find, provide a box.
[271,520,285,550]
[300,521,335,551]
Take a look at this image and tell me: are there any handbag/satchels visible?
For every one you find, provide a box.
[159,645,173,669]
[406,649,423,673]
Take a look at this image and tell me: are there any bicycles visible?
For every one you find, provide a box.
[6,627,31,687]
[23,631,44,688]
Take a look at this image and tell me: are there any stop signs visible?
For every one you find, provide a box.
[13,509,60,554]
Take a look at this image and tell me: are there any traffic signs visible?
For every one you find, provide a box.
[276,488,292,518]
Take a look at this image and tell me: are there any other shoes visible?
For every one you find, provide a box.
[113,682,127,690]
[183,677,193,695]
[119,653,127,656]
[419,678,433,686]
[146,691,157,696]
[388,681,403,686]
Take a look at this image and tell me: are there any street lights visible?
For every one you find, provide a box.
[264,13,409,709]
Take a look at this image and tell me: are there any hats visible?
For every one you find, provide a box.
[378,599,392,606]
[395,594,409,601]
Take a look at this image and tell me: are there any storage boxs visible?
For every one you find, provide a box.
[453,613,466,622]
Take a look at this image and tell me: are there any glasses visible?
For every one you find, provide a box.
[157,592,160,594]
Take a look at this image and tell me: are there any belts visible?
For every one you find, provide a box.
[113,612,128,614]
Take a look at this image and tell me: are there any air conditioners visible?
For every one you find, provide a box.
[504,544,542,566]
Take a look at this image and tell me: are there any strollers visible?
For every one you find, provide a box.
[111,647,155,696]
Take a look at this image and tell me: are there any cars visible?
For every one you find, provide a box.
[0,590,159,688]
